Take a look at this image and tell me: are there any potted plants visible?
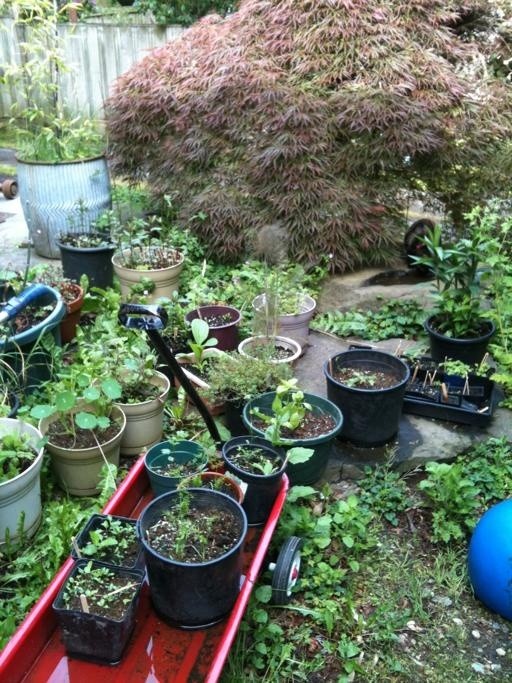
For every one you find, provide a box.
[236,224,302,372]
[33,267,84,346]
[0,417,46,551]
[56,201,117,295]
[411,223,496,371]
[110,219,186,307]
[0,268,65,395]
[252,285,317,358]
[4,64,113,258]
[184,304,241,353]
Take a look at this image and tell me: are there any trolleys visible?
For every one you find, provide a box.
[1,300,305,681]
